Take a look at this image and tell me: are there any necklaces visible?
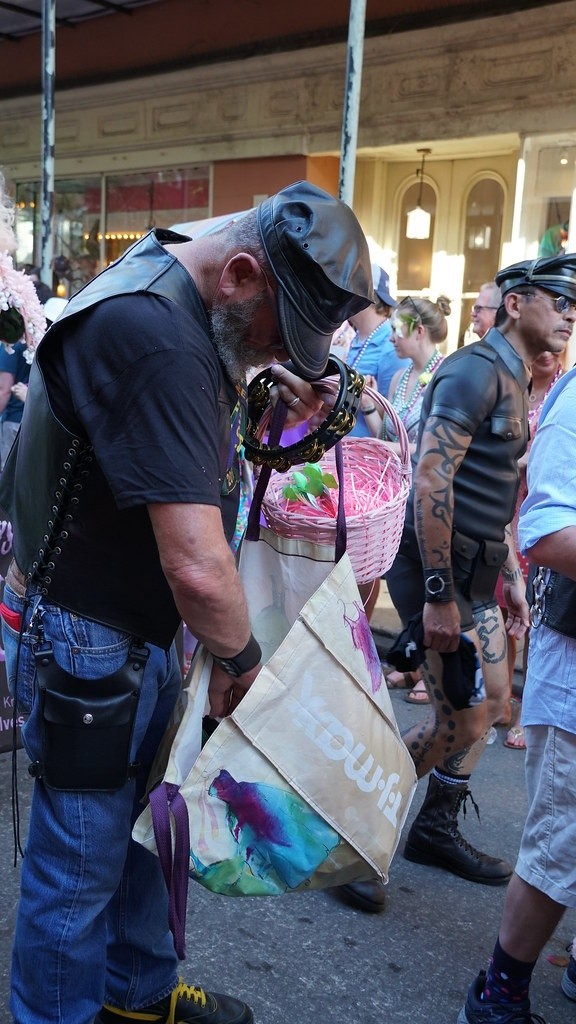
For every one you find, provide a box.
[342,316,391,370]
[361,348,443,442]
[529,376,549,403]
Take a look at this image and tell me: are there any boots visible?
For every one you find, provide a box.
[331,880,386,912]
[405,774,514,886]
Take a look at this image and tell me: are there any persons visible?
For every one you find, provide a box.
[457,364,576,1024]
[322,253,576,908]
[0,258,58,641]
[327,266,565,755]
[1,181,374,1024]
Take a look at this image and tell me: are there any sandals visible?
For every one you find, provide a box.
[504,727,526,749]
[404,689,430,705]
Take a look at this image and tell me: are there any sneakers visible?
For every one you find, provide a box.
[456,969,546,1024]
[560,955,576,996]
[95,981,255,1024]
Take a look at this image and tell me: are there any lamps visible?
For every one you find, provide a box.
[405,148,433,240]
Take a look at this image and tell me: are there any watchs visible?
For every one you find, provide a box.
[502,566,522,581]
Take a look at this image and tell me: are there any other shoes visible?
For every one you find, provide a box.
[383,671,416,689]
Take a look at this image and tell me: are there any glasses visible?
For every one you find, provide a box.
[235,249,287,352]
[528,293,568,313]
[475,306,498,313]
[401,296,421,318]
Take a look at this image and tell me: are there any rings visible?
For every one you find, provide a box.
[288,397,300,407]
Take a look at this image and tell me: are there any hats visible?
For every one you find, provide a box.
[258,180,375,378]
[371,263,397,307]
[495,253,576,300]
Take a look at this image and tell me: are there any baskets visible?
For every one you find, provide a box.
[256,378,412,587]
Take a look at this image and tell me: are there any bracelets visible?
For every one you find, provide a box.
[423,567,459,602]
[361,408,376,415]
[209,632,262,677]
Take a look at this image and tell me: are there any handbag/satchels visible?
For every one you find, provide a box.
[35,646,147,797]
[130,396,418,962]
